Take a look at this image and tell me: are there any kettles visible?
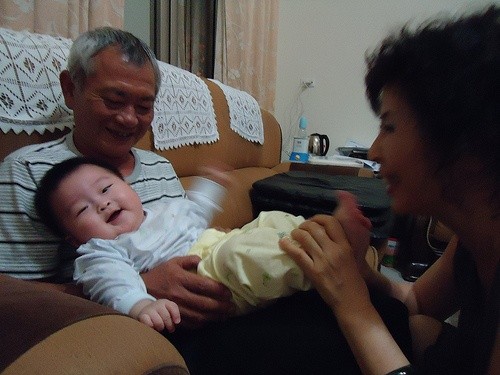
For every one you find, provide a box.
[308,133,330,156]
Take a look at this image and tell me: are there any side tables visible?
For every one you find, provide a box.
[248,167,392,266]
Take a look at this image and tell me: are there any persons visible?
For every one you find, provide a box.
[35,155,373,332]
[280,4,500,375]
[1,25,416,374]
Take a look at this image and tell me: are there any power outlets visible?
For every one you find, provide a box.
[300,78,316,88]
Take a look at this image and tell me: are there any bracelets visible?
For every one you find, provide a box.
[386,363,415,374]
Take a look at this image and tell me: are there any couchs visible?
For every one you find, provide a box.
[0,27,377,375]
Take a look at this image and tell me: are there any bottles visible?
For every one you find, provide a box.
[289,115,310,164]
